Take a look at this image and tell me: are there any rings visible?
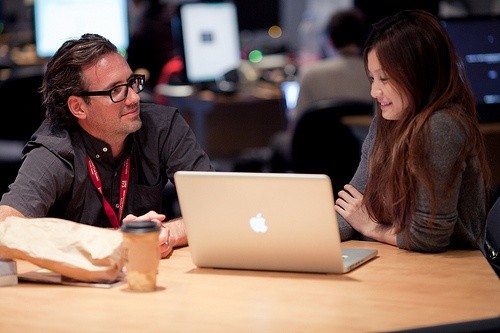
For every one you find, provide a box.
[165,241,169,246]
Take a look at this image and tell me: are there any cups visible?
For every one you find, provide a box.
[121,221,161,291]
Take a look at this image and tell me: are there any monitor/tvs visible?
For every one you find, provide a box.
[170,2,240,92]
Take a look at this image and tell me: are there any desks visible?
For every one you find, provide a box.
[0,240,500,332]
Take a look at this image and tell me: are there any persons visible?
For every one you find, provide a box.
[334,10,493,254]
[294,10,375,122]
[0,33,216,260]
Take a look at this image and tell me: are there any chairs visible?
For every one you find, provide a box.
[291,96,375,204]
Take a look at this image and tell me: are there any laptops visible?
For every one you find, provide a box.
[173,170,378,274]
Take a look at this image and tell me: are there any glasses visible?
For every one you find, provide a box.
[79,73,145,103]
